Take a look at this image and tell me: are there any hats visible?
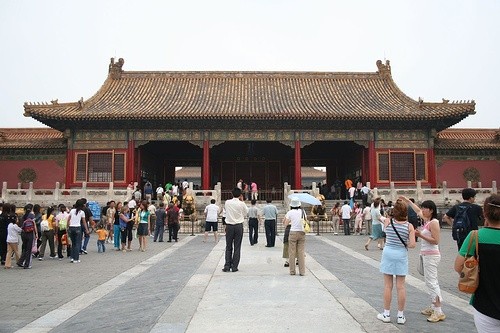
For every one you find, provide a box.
[290,197,302,208]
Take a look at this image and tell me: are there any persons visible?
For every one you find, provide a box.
[236,179,259,201]
[454,194,500,333]
[261,197,279,247]
[330,197,423,251]
[222,188,249,271]
[442,188,485,251]
[247,199,261,246]
[0,177,202,268]
[399,195,446,322]
[219,206,227,235]
[304,173,370,204]
[377,201,416,324]
[282,197,308,276]
[203,198,222,243]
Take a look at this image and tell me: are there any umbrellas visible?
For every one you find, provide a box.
[288,192,323,206]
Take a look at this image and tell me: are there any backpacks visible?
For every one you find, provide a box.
[23,219,35,233]
[452,203,474,240]
[59,213,67,231]
[40,219,50,233]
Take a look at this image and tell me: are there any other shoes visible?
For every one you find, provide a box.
[296,259,298,265]
[222,268,239,272]
[380,247,383,250]
[353,233,360,236]
[0,233,179,270]
[365,244,368,250]
[377,244,380,248]
[284,261,289,267]
[368,232,371,235]
[333,232,338,235]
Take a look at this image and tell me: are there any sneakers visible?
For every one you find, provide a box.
[397,316,407,324]
[377,313,391,323]
[426,312,445,323]
[421,306,434,315]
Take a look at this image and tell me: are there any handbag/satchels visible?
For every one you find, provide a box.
[31,237,38,254]
[417,254,424,275]
[61,233,72,246]
[457,230,480,293]
[301,209,310,234]
